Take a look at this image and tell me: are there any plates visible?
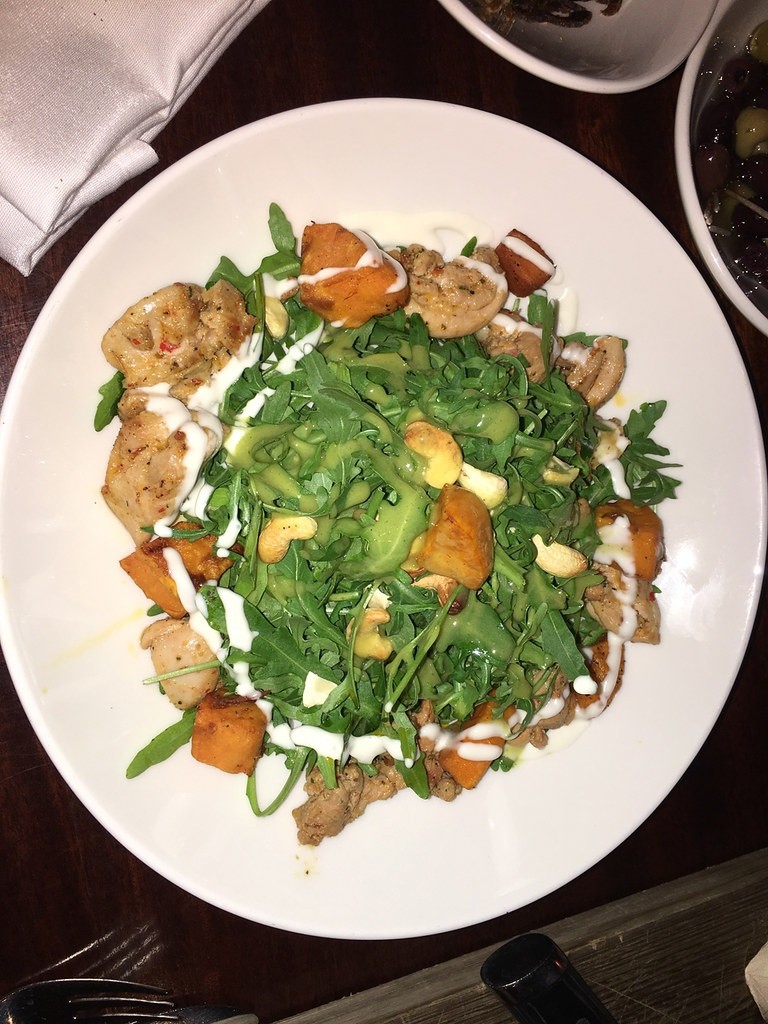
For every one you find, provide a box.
[0,97,768,942]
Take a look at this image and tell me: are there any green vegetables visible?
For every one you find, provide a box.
[93,204,678,813]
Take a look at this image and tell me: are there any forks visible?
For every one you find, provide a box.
[0,978,179,1024]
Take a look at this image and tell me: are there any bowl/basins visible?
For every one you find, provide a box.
[437,0,719,95]
[674,0,768,338]
